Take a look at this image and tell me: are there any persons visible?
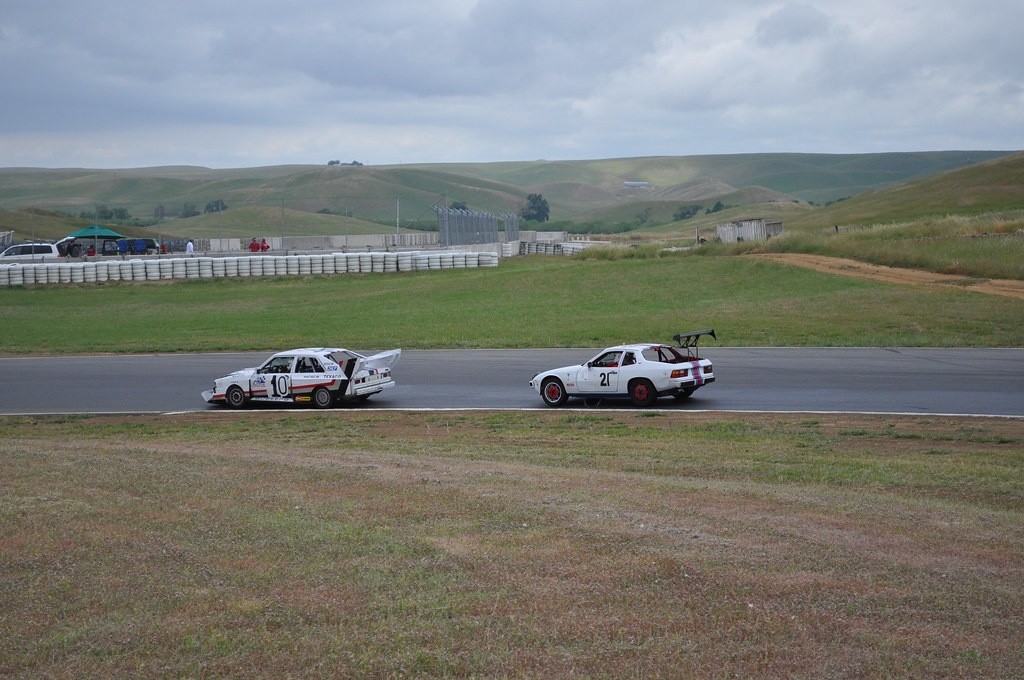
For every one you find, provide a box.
[186,239,194,258]
[67,243,82,257]
[170,240,175,254]
[249,238,260,252]
[261,239,270,252]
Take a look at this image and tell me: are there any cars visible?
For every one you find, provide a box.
[0,237,75,260]
[201,347,402,411]
[531,329,716,409]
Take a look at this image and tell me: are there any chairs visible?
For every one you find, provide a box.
[296,360,306,373]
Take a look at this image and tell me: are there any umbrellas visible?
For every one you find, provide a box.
[63,224,126,255]
